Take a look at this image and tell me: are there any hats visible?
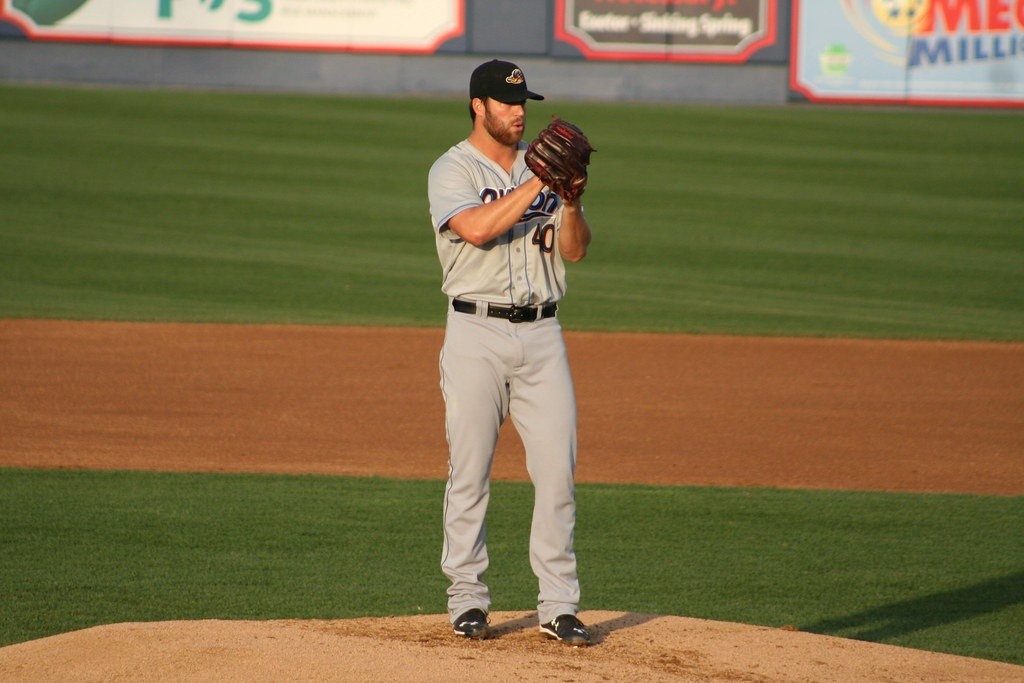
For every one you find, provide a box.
[469,59,545,103]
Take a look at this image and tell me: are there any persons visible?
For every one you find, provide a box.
[427,61,595,646]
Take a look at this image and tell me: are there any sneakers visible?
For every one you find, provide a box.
[453,609,496,639]
[539,614,591,645]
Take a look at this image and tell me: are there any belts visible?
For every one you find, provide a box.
[452,299,557,323]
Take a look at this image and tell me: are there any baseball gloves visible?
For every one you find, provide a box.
[523,119,593,202]
